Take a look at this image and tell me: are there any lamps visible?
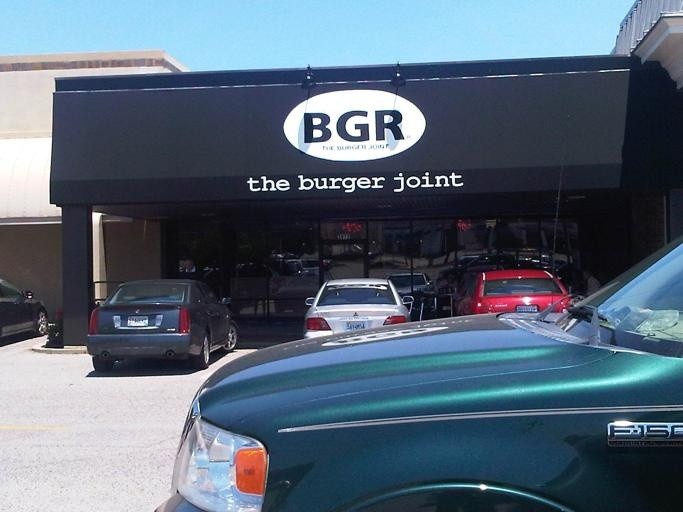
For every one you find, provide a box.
[391,64,406,87]
[302,66,316,89]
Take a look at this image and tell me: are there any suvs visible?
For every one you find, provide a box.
[152,229,682,512]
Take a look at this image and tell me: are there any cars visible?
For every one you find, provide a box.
[301,273,417,339]
[85,281,238,373]
[454,266,571,319]
[0,279,47,346]
